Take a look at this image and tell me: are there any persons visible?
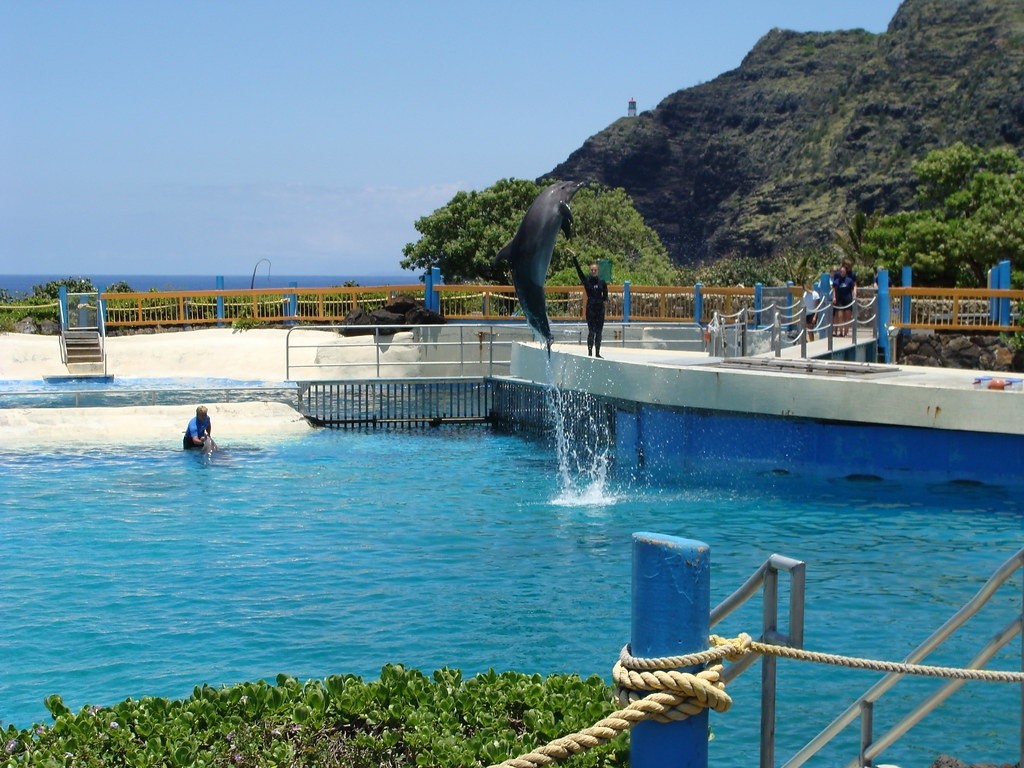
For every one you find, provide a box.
[183,406,212,450]
[873,266,893,288]
[802,281,820,341]
[566,248,608,358]
[820,259,857,337]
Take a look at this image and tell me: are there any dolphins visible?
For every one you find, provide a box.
[495,181,586,361]
[199,429,219,469]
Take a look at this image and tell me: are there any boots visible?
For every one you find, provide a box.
[588,343,593,356]
[595,342,603,358]
